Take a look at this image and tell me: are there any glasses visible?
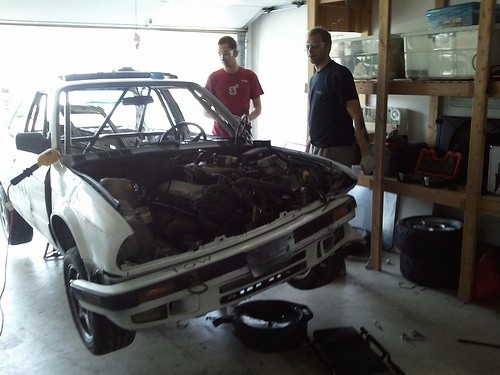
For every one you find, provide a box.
[218,48,236,60]
[306,41,327,50]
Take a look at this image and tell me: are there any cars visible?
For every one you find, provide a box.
[0,66,360,356]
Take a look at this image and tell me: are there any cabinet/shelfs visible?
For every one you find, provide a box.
[306,0,500,303]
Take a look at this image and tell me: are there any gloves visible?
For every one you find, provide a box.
[360,155,375,173]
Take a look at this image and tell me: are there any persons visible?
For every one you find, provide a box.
[202,36,264,137]
[304,27,375,276]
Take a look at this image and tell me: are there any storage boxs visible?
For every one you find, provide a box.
[425,1,500,31]
[327,33,407,80]
[399,24,500,80]
